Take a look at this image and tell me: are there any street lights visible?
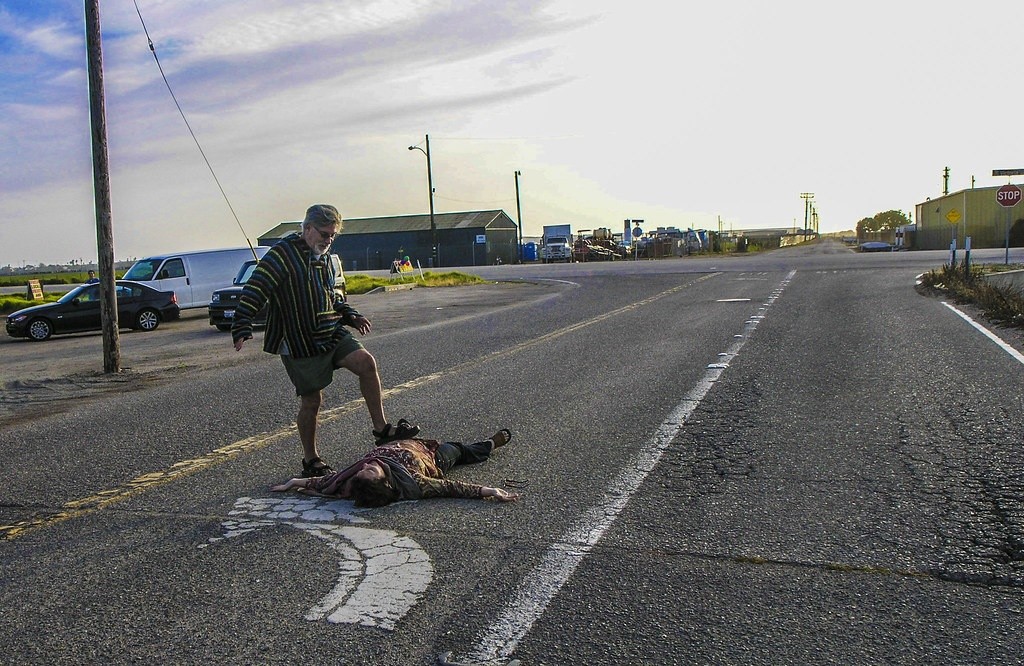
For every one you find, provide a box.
[515,170,525,265]
[408,147,438,267]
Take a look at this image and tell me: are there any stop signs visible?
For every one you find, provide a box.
[995,185,1022,208]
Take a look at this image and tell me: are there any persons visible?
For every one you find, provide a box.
[230,204,420,478]
[271,417,519,509]
[81,270,100,301]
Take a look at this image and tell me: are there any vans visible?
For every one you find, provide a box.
[118,247,272,311]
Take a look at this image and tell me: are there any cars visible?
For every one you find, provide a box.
[5,275,180,340]
[618,241,630,249]
[208,256,270,334]
[634,237,654,248]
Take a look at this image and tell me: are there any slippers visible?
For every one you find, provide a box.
[487,429,511,450]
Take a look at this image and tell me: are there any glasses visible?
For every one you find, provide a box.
[310,224,338,239]
[503,477,530,489]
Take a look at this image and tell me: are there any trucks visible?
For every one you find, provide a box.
[540,225,573,263]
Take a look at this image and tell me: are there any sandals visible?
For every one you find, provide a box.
[371,418,421,447]
[301,457,334,478]
